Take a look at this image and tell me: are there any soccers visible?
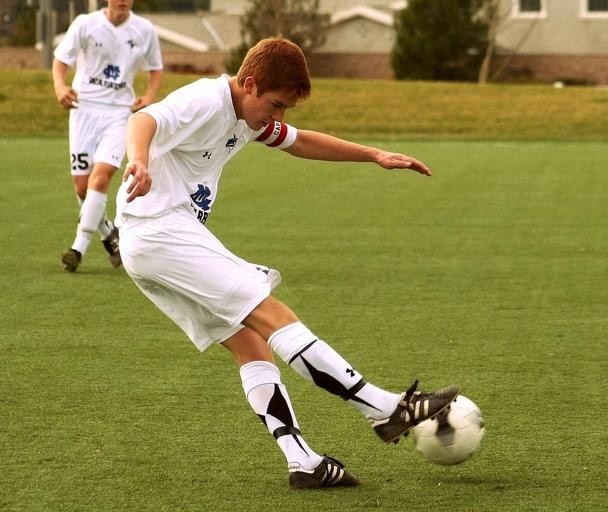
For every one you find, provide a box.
[414,395,483,464]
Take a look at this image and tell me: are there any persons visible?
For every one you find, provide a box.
[114,37,462,488]
[52,0,163,272]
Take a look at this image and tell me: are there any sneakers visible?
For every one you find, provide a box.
[103,227,121,268]
[287,454,360,490]
[61,247,82,272]
[373,380,462,444]
